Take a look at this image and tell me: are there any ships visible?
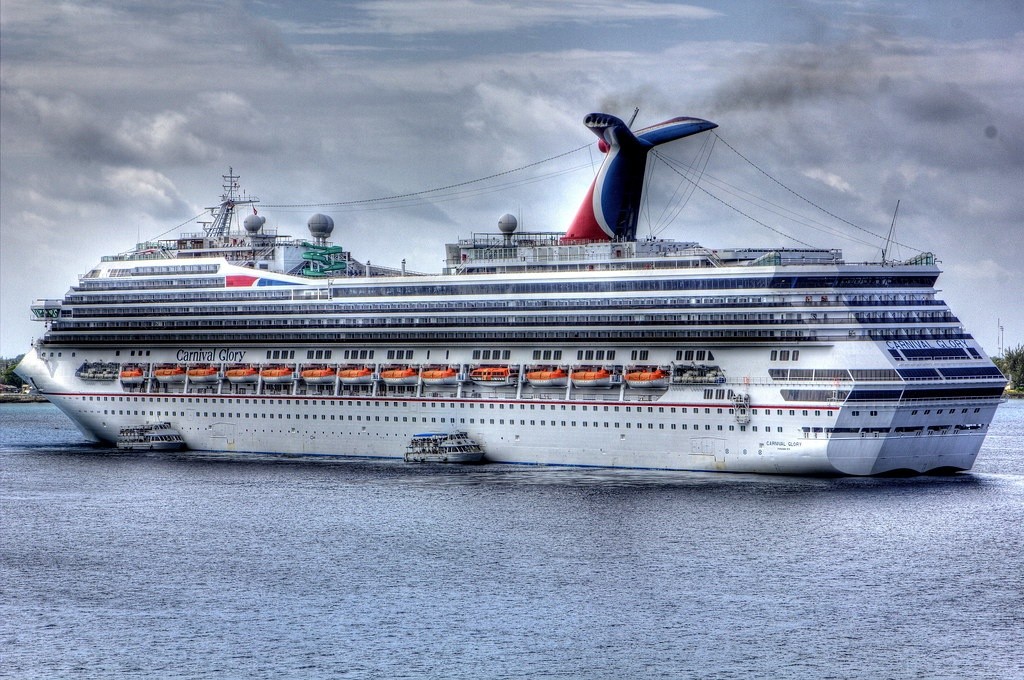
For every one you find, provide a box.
[12,106,1008,488]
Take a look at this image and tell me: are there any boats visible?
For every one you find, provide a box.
[119,363,670,388]
[402,430,485,464]
[115,422,186,453]
